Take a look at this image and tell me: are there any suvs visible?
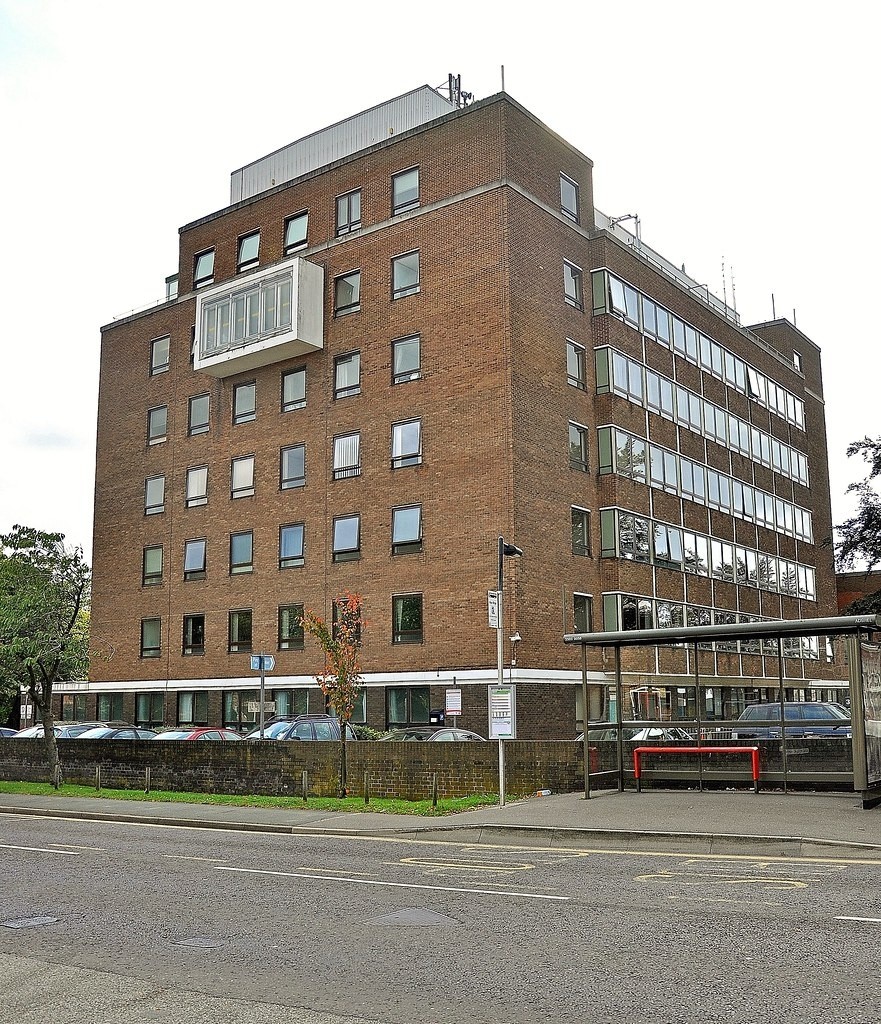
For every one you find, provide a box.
[732,701,852,739]
[241,713,358,741]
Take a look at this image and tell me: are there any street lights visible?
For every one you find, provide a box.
[496,534,523,808]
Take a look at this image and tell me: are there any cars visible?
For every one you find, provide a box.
[377,726,487,741]
[150,728,243,740]
[76,728,159,740]
[28,726,97,739]
[574,728,694,740]
